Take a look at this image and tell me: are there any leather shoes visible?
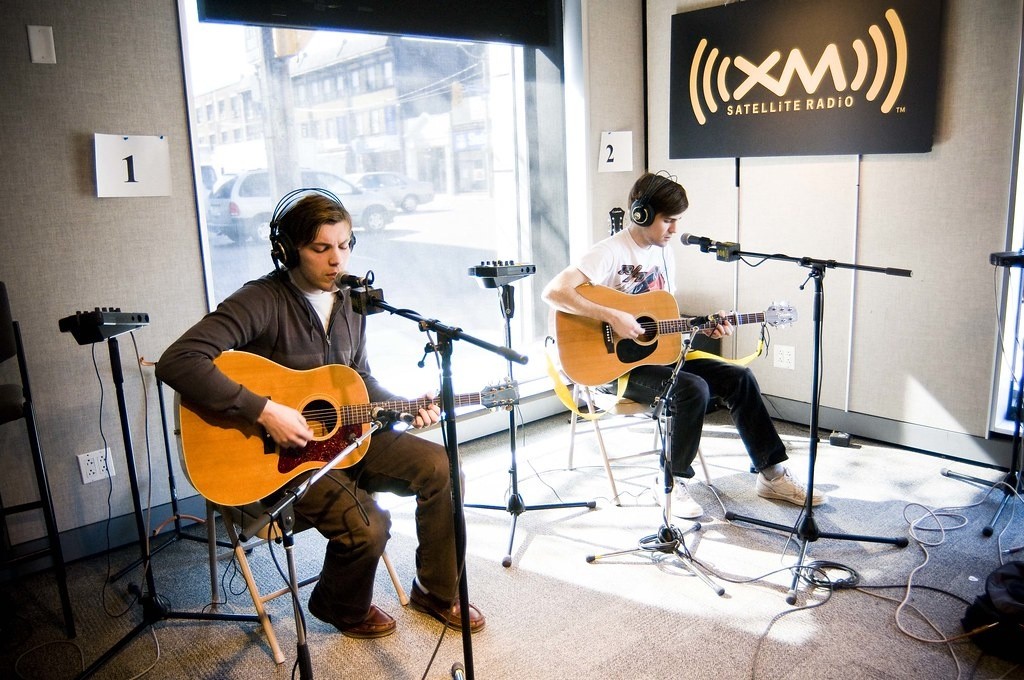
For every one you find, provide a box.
[308,597,395,639]
[410,577,484,633]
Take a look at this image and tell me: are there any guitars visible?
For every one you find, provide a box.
[174,350,520,507]
[549,283,799,387]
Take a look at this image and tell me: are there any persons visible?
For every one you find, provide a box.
[540,174,828,519]
[155,190,486,634]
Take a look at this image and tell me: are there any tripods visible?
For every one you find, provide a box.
[941,267,1023,536]
[75,338,271,680]
[462,285,596,567]
[700,237,913,605]
[586,317,726,597]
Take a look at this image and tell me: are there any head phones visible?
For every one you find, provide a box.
[269,188,356,269]
[631,170,677,227]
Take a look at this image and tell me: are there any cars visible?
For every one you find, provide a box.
[346,170,437,214]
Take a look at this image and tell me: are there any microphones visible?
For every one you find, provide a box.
[681,233,727,247]
[687,314,720,327]
[334,270,372,290]
[270,250,300,298]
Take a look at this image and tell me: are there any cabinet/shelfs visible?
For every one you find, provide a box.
[0,279,77,641]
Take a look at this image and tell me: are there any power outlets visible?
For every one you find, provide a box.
[75,446,117,485]
[773,344,796,370]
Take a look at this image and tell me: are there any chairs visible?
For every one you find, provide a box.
[205,499,409,663]
[565,382,711,506]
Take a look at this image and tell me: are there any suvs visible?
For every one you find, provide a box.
[207,168,400,243]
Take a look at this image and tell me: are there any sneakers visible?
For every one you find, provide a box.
[757,467,828,507]
[654,477,703,518]
